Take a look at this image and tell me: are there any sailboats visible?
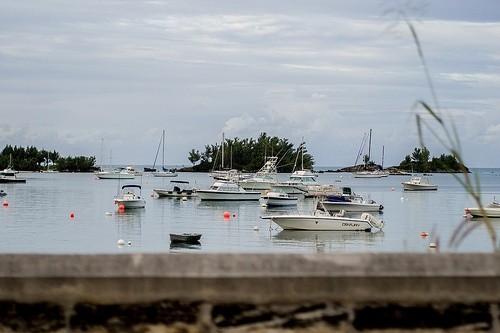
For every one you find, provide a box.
[151,130,178,177]
[95,136,135,179]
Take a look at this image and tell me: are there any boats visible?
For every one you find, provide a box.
[314,187,383,212]
[466,196,500,218]
[0,165,26,182]
[169,232,201,242]
[154,180,200,196]
[302,180,351,196]
[196,148,294,190]
[260,198,385,230]
[354,169,390,178]
[259,136,322,195]
[401,168,438,190]
[196,170,261,201]
[115,174,145,209]
